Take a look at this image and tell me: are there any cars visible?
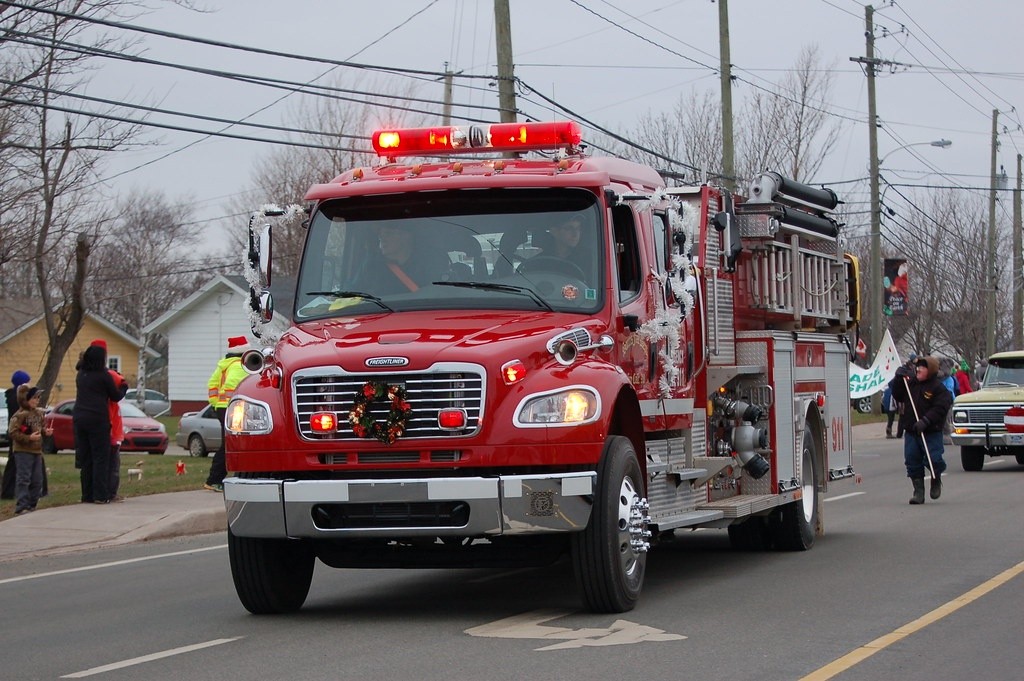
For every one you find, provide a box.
[849,359,885,413]
[0,388,13,448]
[41,399,169,455]
[122,388,168,417]
[174,402,222,458]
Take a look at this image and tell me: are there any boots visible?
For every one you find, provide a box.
[930,469,941,498]
[908,475,925,503]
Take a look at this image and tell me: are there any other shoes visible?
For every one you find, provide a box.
[94,493,125,505]
[204,482,223,492]
[886,434,895,438]
[897,433,902,438]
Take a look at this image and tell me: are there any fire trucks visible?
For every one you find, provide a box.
[222,121,862,616]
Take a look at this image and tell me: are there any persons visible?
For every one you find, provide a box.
[882,380,898,439]
[522,210,592,284]
[0,370,48,500]
[203,336,251,492]
[8,383,54,515]
[73,340,129,503]
[891,357,952,504]
[358,220,448,296]
[895,354,988,444]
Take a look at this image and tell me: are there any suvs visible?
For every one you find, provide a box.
[950,351,1024,471]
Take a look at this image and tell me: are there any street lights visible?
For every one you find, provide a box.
[869,137,952,414]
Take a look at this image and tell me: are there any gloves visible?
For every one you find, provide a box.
[913,416,930,436]
[894,367,906,381]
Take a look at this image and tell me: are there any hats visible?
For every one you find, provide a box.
[910,354,916,360]
[227,336,250,354]
[10,370,30,386]
[960,359,971,372]
[32,389,45,399]
